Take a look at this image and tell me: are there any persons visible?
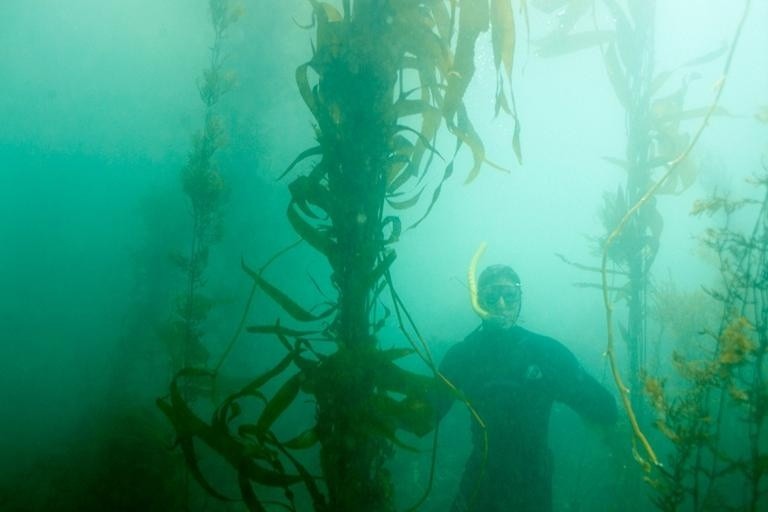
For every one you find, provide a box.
[399,264,617,512]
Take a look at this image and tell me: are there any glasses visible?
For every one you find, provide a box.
[476,285,521,305]
